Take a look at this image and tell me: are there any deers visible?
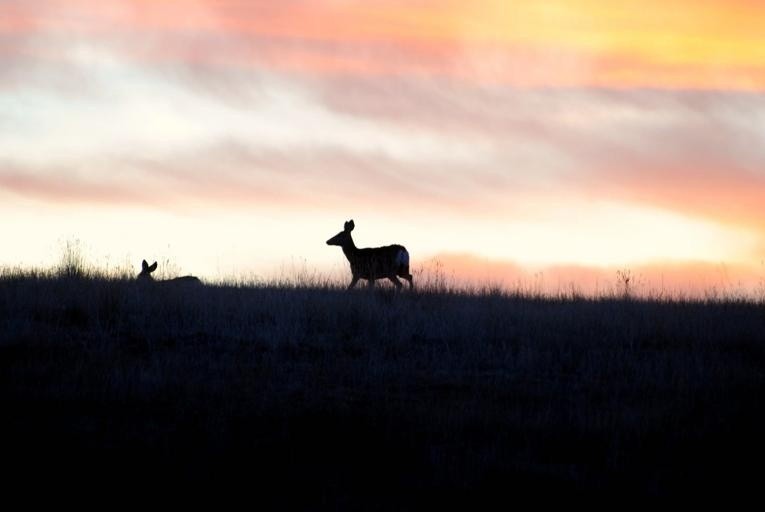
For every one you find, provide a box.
[325,219,414,293]
[135,259,203,287]
